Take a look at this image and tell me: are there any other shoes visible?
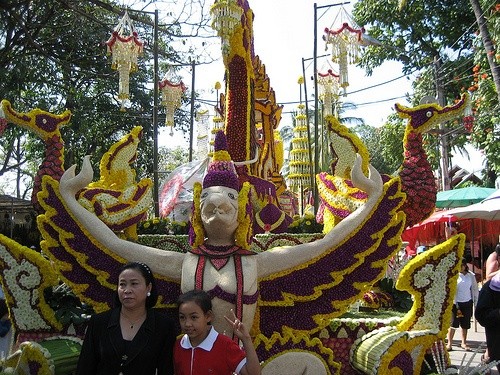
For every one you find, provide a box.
[448,348,452,351]
[482,355,492,375]
[461,344,472,352]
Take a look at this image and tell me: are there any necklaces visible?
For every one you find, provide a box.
[121,310,144,329]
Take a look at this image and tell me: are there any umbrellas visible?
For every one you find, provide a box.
[400,184,500,286]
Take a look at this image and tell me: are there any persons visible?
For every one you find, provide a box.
[474,234,500,375]
[75,262,181,375]
[58,127,383,374]
[173,289,262,375]
[445,257,478,351]
